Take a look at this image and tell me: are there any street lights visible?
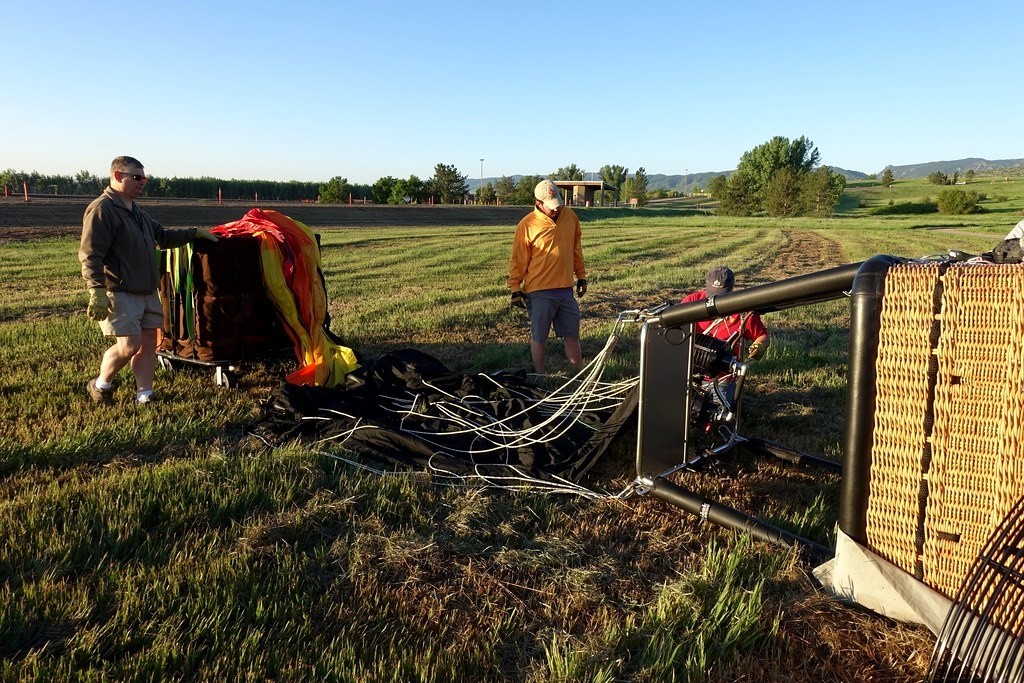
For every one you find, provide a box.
[479,158,485,197]
[683,168,689,194]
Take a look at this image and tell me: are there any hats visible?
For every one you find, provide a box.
[534,180,563,209]
[706,266,735,296]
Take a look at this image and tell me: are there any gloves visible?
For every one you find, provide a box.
[511,291,530,309]
[747,342,765,361]
[194,227,221,243]
[577,279,587,297]
[86,287,114,321]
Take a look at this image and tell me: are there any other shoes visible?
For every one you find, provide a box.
[134,392,161,406]
[86,379,115,408]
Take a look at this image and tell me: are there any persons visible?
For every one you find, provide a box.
[509,180,588,374]
[681,267,770,406]
[78,156,219,407]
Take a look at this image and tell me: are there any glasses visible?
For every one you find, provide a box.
[121,173,147,183]
